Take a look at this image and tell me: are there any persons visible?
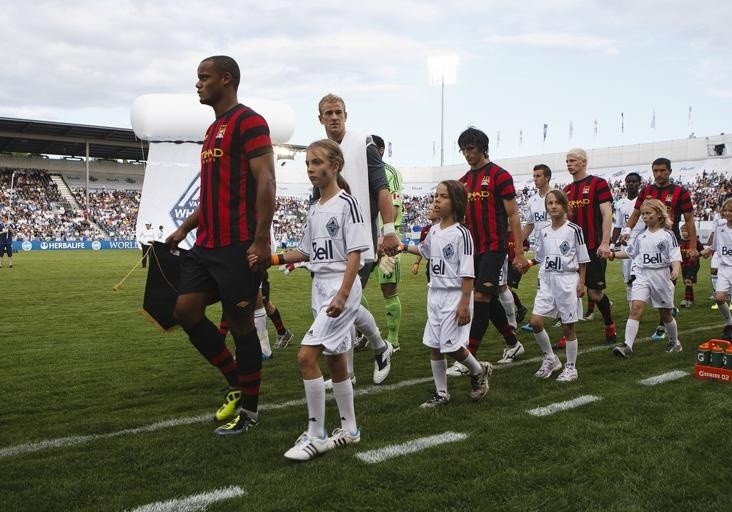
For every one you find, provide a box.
[351,133,405,352]
[677,222,705,308]
[519,163,562,332]
[620,158,698,342]
[216,269,294,351]
[610,171,647,312]
[599,197,683,359]
[384,178,494,411]
[0,215,15,268]
[702,191,732,335]
[312,92,399,290]
[552,147,617,350]
[280,231,289,252]
[250,285,274,359]
[163,53,278,438]
[444,125,527,377]
[412,201,434,282]
[491,252,517,335]
[399,237,415,246]
[507,239,528,323]
[516,188,587,383]
[243,138,375,464]
[582,293,600,323]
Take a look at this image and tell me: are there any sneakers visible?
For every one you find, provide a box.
[551,336,567,349]
[211,387,245,423]
[325,428,362,450]
[533,355,563,380]
[663,342,686,355]
[323,376,357,392]
[611,342,633,359]
[387,341,402,355]
[496,341,526,364]
[604,320,617,343]
[271,330,294,350]
[651,324,669,340]
[213,410,260,435]
[728,302,732,310]
[468,360,494,403]
[417,392,453,408]
[283,431,329,461]
[556,361,579,381]
[354,325,384,352]
[514,304,528,324]
[679,298,697,309]
[581,311,595,321]
[552,318,562,329]
[522,321,534,333]
[710,303,718,309]
[446,360,473,378]
[709,294,714,300]
[372,339,394,384]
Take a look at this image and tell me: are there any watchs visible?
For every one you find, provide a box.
[415,261,420,265]
[612,250,616,259]
[531,258,536,266]
[698,251,702,257]
[404,244,408,252]
[278,253,284,265]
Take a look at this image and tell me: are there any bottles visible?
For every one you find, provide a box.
[709,345,723,368]
[697,343,710,366]
[724,345,732,371]
